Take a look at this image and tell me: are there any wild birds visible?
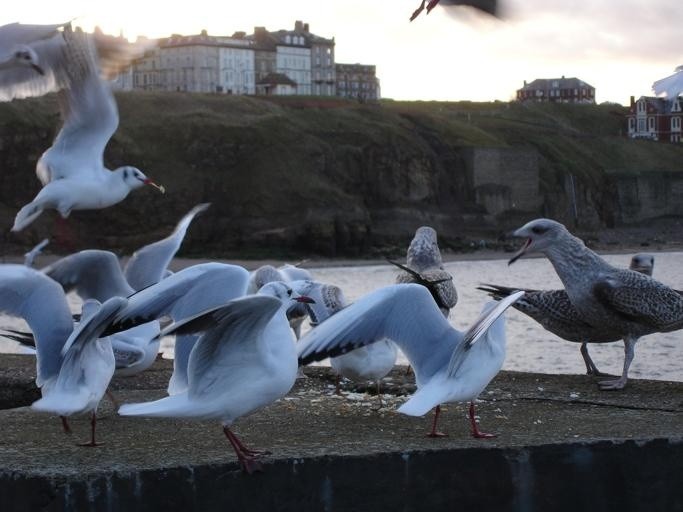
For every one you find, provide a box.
[2,25,163,236]
[0,227,523,471]
[408,2,513,30]
[479,217,682,390]
